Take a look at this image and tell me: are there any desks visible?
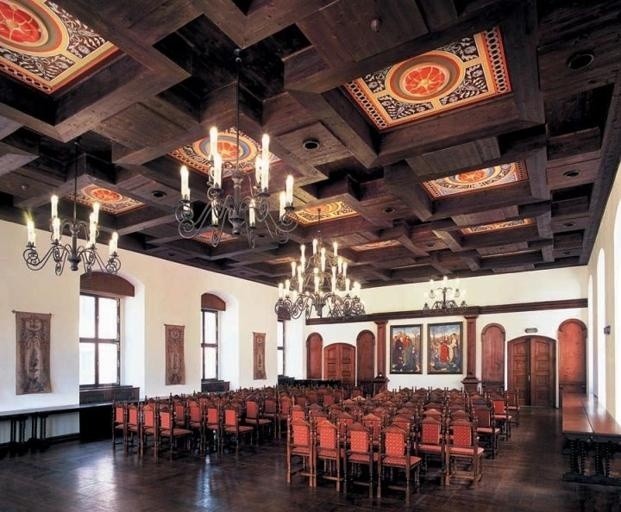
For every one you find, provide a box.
[560,386,621,490]
[1,401,108,458]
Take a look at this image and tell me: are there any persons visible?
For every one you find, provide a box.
[393,329,419,371]
[431,332,462,371]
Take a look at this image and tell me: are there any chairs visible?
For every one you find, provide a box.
[109,383,525,511]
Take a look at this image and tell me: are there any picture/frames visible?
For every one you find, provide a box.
[426,321,465,376]
[389,323,424,376]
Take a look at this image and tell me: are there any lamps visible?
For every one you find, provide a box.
[167,43,303,251]
[18,136,126,279]
[419,271,472,318]
[273,230,369,323]
